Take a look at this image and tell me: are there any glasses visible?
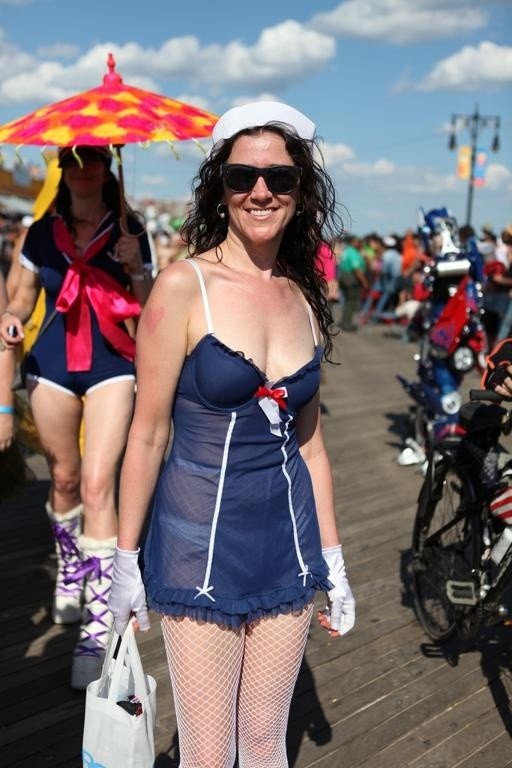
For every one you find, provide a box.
[220,160,303,195]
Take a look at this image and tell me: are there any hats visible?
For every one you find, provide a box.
[210,101,315,149]
[57,145,114,167]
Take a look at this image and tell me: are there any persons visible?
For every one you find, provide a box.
[392,204,481,475]
[321,231,511,352]
[0,274,18,449]
[1,215,197,389]
[0,146,156,691]
[108,98,359,768]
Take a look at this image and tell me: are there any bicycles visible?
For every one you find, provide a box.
[398,386,512,734]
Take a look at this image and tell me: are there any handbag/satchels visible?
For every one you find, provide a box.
[82,667,159,767]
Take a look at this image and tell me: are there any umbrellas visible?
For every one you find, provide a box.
[0,48,218,234]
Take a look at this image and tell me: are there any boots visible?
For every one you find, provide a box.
[44,501,85,626]
[72,534,119,690]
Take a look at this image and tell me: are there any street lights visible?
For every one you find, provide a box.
[446,101,501,225]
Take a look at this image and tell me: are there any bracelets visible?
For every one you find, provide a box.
[0,406,14,415]
